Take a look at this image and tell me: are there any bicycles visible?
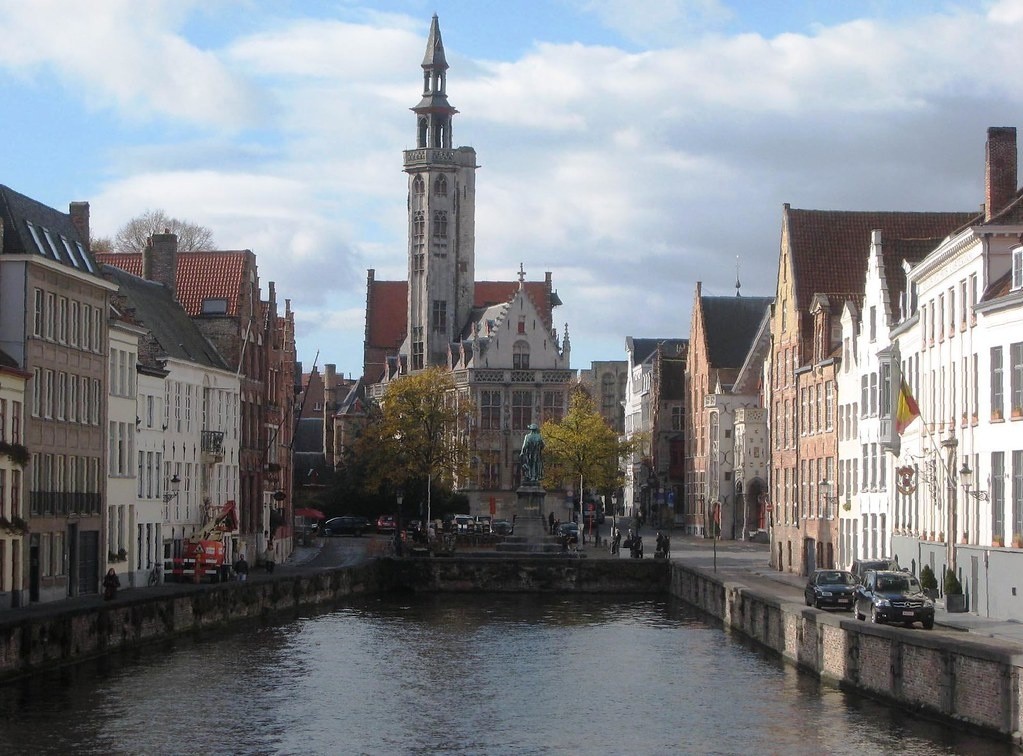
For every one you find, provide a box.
[147,560,164,587]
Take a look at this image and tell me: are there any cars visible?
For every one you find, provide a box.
[451,512,513,535]
[804,568,857,610]
[557,522,578,543]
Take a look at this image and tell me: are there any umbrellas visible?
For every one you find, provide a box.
[295,507,325,545]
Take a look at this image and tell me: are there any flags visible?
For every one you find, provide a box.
[895,371,921,436]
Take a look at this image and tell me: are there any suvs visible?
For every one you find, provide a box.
[853,568,935,631]
[375,514,399,535]
[852,556,909,586]
[311,516,367,537]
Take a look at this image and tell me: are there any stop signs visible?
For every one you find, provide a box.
[767,504,773,513]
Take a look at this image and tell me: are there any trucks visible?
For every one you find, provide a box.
[573,500,599,528]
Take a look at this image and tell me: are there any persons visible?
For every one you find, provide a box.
[656,530,670,558]
[612,525,621,554]
[103,568,121,601]
[627,528,643,559]
[412,520,436,542]
[264,543,276,576]
[548,511,561,536]
[234,554,249,588]
[519,423,545,483]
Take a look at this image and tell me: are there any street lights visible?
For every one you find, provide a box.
[633,497,642,537]
[960,463,974,543]
[396,492,405,555]
[819,478,830,515]
[609,492,619,554]
